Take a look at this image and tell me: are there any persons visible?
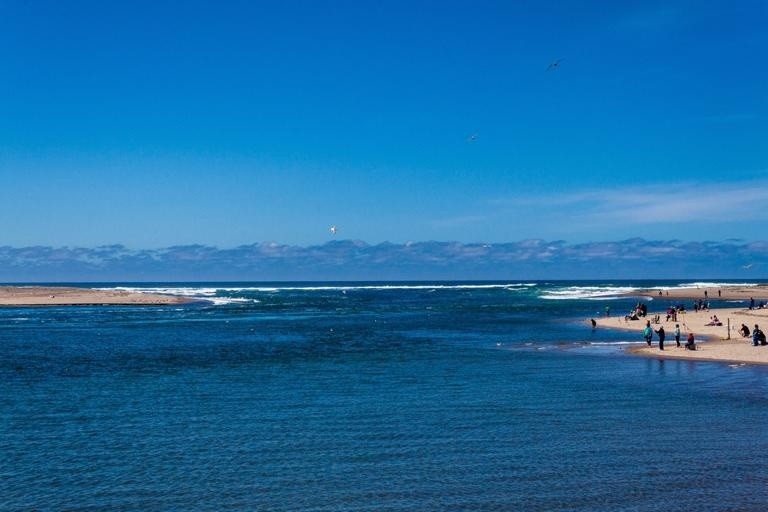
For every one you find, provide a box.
[739,324,768,346]
[749,297,763,309]
[659,291,669,295]
[604,304,610,316]
[709,315,722,326]
[704,290,707,296]
[666,302,685,321]
[626,302,647,320]
[591,319,596,327]
[693,299,710,311]
[644,320,694,350]
[718,289,721,296]
